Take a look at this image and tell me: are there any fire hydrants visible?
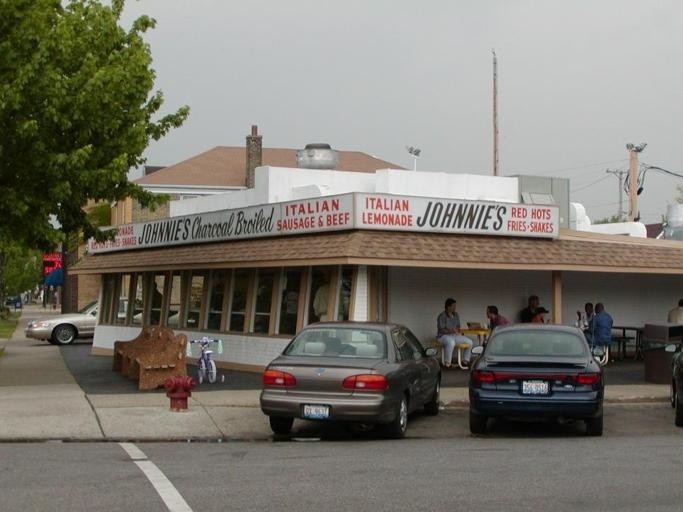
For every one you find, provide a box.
[164,373,196,411]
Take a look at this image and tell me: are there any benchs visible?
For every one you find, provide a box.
[114,323,186,389]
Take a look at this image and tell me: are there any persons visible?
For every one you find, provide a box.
[576,302,613,345]
[487,306,511,329]
[668,298,683,324]
[437,298,473,367]
[519,294,549,323]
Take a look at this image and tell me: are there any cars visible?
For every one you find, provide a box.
[25,296,180,344]
[468,322,603,437]
[664,337,683,427]
[260,321,441,439]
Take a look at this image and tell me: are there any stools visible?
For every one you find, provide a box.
[609,336,635,363]
[432,342,474,370]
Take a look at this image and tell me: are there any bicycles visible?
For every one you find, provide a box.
[191,336,225,384]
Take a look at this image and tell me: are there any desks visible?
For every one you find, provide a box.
[611,326,638,358]
[460,329,492,357]
